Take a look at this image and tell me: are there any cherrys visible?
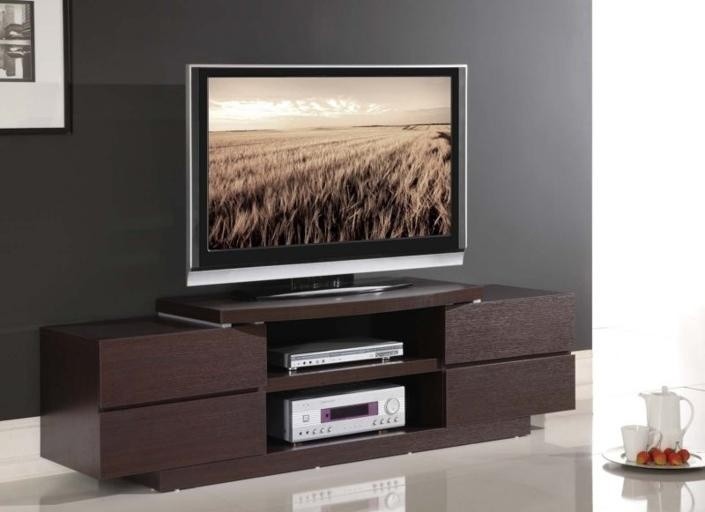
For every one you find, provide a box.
[637,440,690,465]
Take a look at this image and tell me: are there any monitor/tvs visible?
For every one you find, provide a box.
[183,61,471,302]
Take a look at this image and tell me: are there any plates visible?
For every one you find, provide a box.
[601,444,705,472]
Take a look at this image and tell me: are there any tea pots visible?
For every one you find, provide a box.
[639,385,695,452]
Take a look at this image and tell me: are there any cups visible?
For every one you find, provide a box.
[620,425,661,463]
